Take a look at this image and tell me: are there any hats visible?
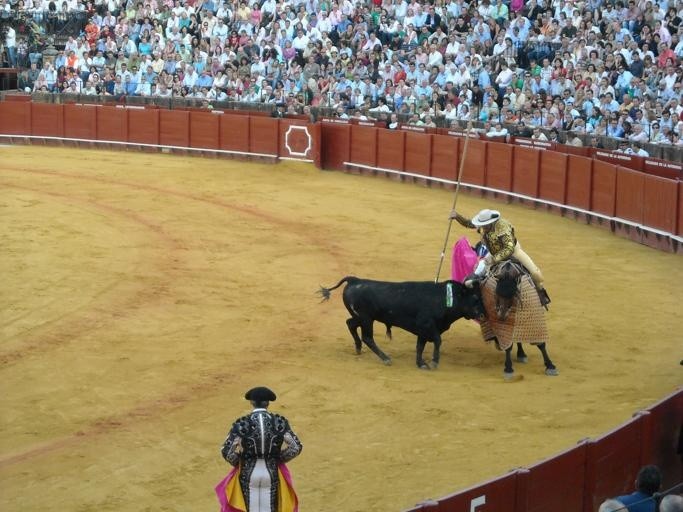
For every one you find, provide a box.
[471,209,500,226]
[245,387,275,401]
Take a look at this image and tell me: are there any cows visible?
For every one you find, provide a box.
[314,276,487,370]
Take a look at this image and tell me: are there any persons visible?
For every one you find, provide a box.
[445,206,547,306]
[221,386,303,512]
[598,464,683,512]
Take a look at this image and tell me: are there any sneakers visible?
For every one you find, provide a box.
[540,288,550,304]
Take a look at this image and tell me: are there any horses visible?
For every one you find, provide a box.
[475,241,559,379]
